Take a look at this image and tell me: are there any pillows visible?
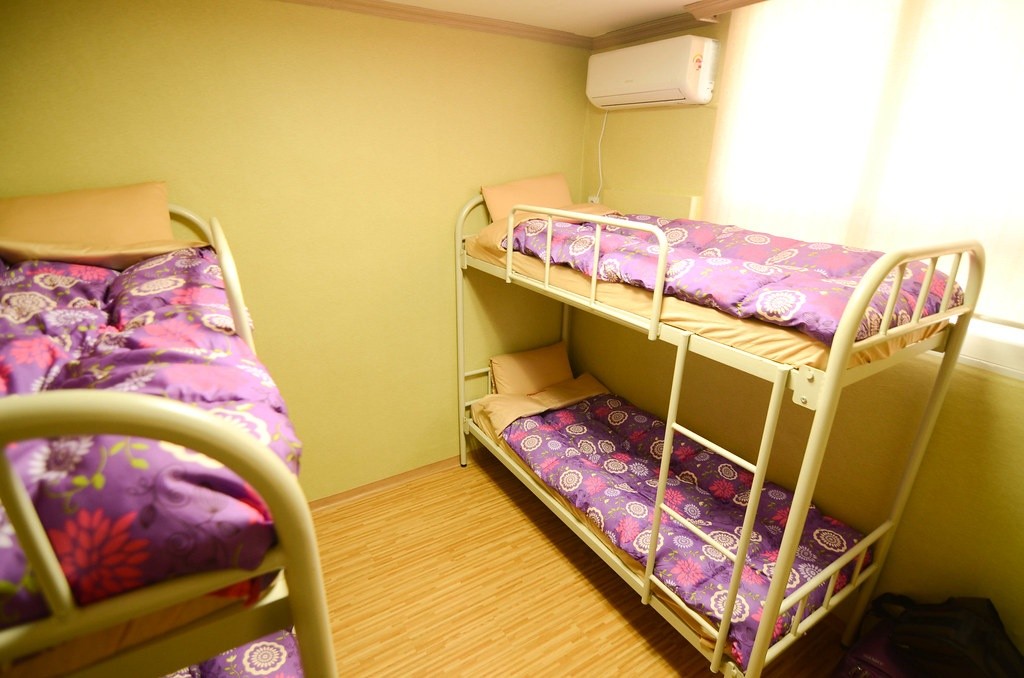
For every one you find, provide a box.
[0,179,212,272]
[490,341,574,395]
[480,172,574,226]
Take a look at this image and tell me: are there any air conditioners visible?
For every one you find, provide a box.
[584,34,719,113]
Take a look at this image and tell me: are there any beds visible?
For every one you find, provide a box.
[454,194,985,412]
[0,208,335,677]
[458,365,895,678]
